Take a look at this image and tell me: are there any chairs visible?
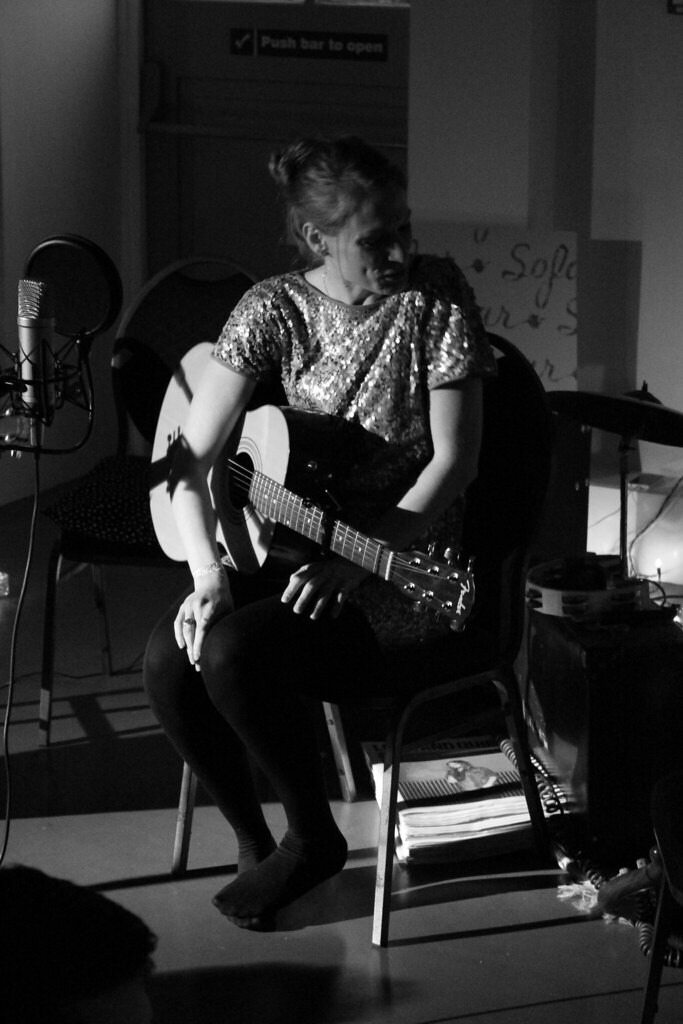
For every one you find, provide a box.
[634,777,683,1024]
[169,331,571,949]
[37,257,259,751]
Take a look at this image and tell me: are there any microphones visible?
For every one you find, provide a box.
[15,277,55,417]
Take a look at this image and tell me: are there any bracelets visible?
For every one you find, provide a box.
[192,561,225,576]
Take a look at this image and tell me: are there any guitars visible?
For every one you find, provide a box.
[147,339,480,634]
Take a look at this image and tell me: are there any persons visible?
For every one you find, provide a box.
[140,134,497,928]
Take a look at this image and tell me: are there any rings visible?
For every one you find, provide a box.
[182,618,195,625]
